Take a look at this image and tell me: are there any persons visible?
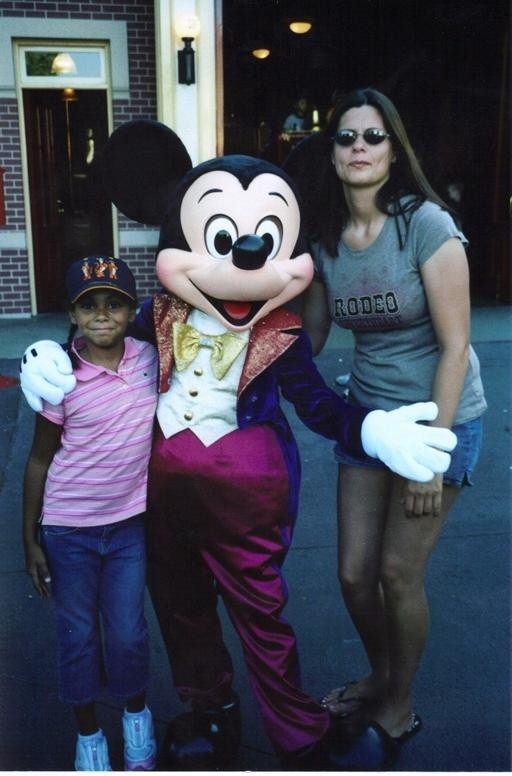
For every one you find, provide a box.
[282,95,308,130]
[298,84,488,763]
[21,253,161,770]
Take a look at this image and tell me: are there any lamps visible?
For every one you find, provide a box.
[170,10,201,86]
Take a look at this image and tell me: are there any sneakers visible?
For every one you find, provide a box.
[73,726,113,771]
[120,702,159,771]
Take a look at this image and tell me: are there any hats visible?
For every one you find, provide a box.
[63,253,139,306]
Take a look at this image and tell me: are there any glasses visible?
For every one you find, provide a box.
[332,126,392,147]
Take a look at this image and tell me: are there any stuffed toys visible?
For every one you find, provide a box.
[19,117,458,771]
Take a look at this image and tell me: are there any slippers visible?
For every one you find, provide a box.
[319,679,376,720]
[366,709,426,748]
